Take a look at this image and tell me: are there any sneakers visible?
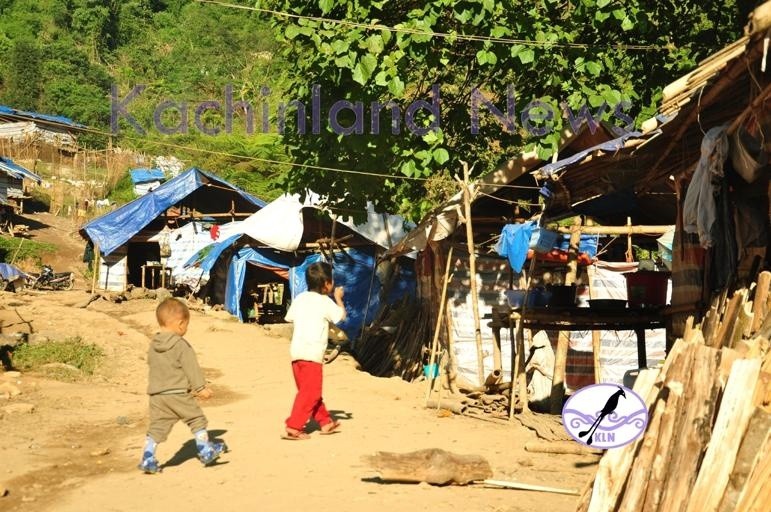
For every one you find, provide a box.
[197,442,225,464]
[138,452,162,474]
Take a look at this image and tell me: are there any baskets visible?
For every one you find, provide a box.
[529,227,559,254]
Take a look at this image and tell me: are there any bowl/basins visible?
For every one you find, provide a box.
[627,301,657,312]
[503,287,552,311]
[540,284,579,303]
[585,297,628,308]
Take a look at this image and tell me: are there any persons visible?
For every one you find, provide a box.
[285,262,348,439]
[136,297,227,473]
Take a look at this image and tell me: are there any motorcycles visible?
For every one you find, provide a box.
[33,264,76,292]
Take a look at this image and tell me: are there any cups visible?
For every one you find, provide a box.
[423,363,438,379]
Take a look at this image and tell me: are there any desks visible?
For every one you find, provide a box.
[480,300,696,414]
[140,265,173,290]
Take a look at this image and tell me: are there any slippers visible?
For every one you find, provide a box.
[320,422,340,434]
[281,430,310,440]
